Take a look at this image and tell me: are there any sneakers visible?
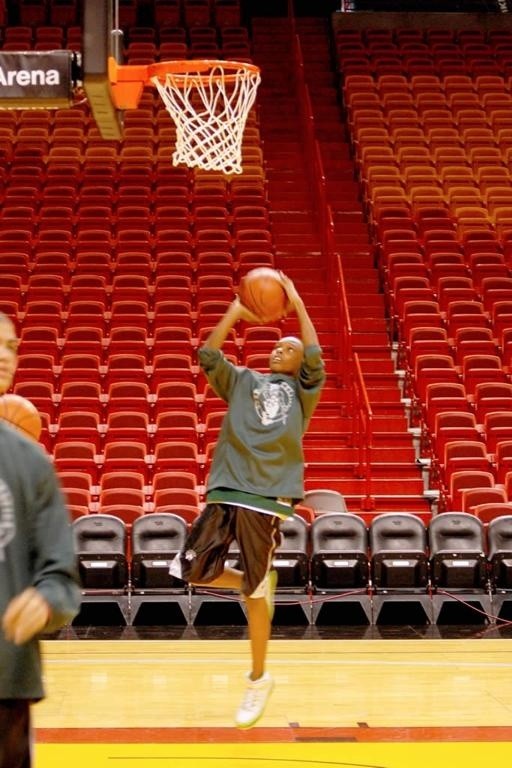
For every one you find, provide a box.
[234,670,274,730]
[266,568,278,620]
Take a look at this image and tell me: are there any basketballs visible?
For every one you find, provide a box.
[240,267,288,322]
[0,394,41,441]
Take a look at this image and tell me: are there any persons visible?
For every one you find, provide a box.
[1,308,85,766]
[167,267,328,728]
